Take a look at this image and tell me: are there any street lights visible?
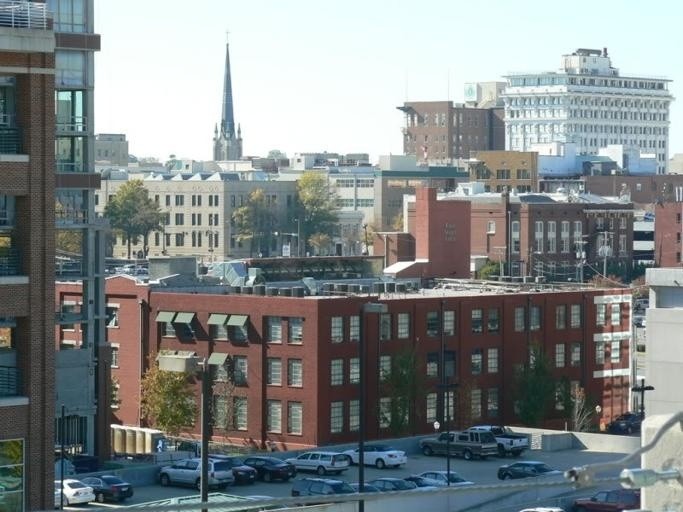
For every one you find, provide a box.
[631,377,654,420]
[356,301,389,510]
[295,219,301,257]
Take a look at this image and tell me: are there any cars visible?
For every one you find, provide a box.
[574,488,641,511]
[81,475,134,504]
[53,478,97,507]
[606,412,642,434]
[633,316,646,327]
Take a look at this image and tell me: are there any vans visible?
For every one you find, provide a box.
[123,264,137,274]
[634,299,649,311]
